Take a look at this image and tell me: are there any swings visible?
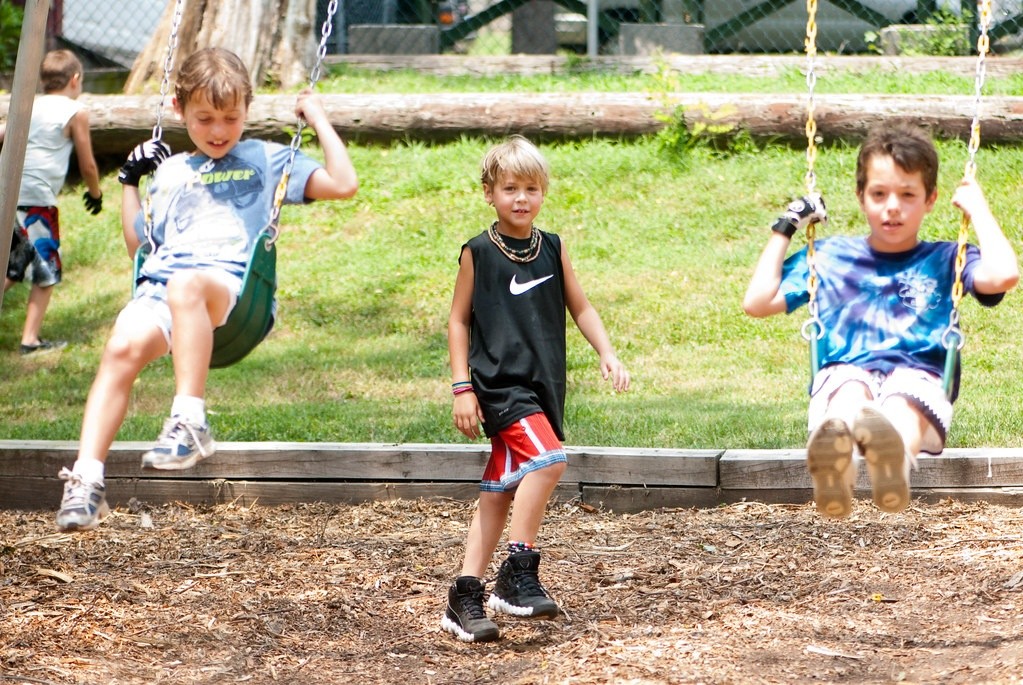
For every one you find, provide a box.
[804,0,990,405]
[129,0,342,370]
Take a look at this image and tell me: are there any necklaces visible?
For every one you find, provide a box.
[486,221,543,264]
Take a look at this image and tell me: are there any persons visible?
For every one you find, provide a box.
[441,131,633,642]
[57,43,358,533]
[740,121,1023,520]
[0,46,106,357]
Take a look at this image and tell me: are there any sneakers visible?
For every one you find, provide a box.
[57,465,111,531]
[488,550,557,621]
[439,576,500,643]
[143,415,217,470]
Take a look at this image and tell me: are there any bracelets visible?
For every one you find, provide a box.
[451,381,474,395]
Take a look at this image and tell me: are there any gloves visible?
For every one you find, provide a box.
[119,139,171,188]
[772,197,826,240]
[83,190,103,215]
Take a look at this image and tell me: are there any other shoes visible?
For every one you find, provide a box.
[858,406,910,513]
[20,337,67,355]
[808,419,856,516]
